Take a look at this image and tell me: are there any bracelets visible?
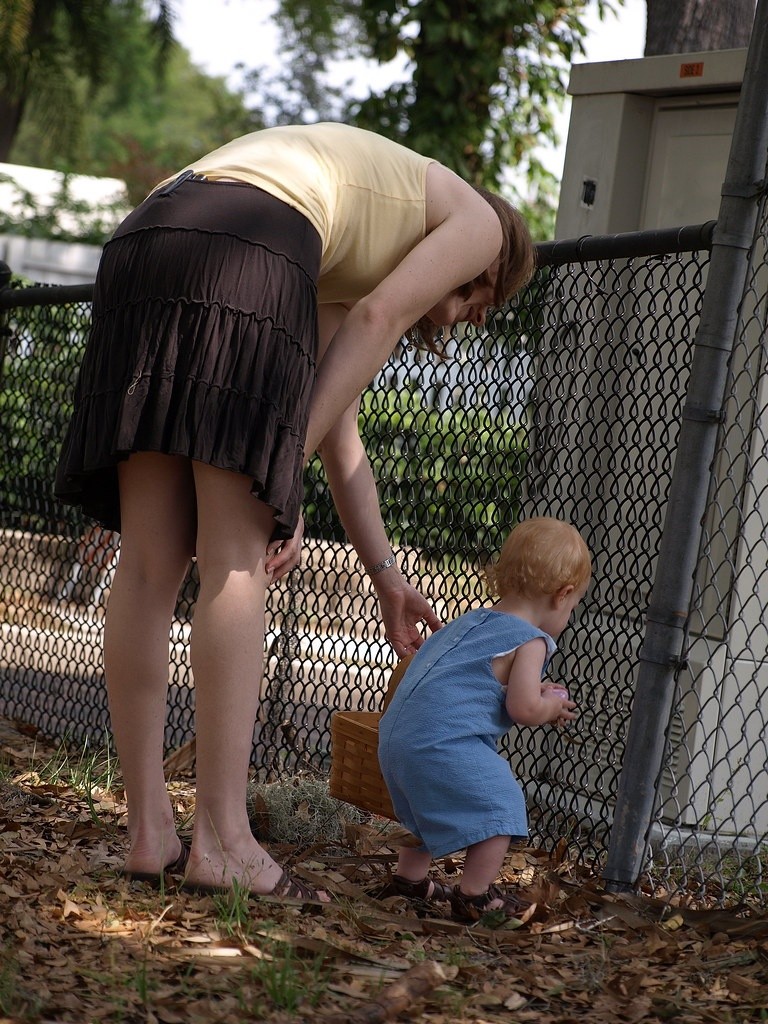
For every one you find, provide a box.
[365,554,397,578]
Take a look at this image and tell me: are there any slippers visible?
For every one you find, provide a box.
[189,871,331,912]
[119,841,191,888]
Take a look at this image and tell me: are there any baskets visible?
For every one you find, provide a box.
[328,655,414,824]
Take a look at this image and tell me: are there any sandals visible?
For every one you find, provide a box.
[451,884,547,928]
[386,875,452,904]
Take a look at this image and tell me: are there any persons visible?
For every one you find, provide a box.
[49,121,534,902]
[377,517,592,925]
